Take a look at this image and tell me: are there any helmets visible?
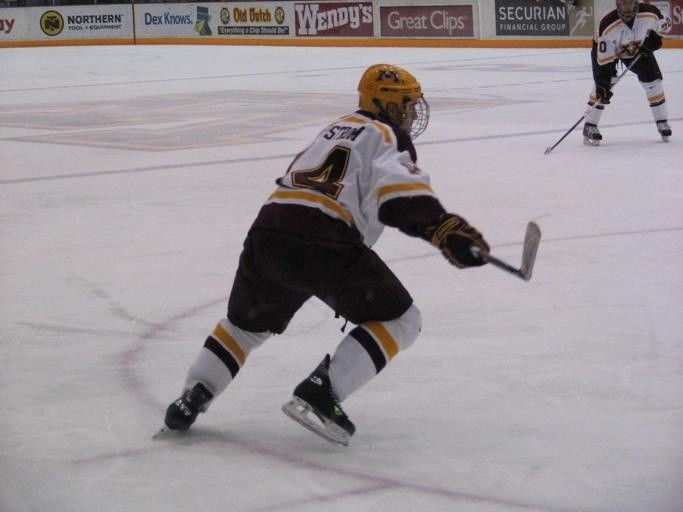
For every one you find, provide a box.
[616,0,638,22]
[358,63,430,143]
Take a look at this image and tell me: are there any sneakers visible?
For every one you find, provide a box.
[164,383,215,431]
[583,122,602,140]
[656,119,672,136]
[292,353,356,436]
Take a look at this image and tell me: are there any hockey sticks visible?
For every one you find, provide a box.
[543,55,641,155]
[469,222,540,281]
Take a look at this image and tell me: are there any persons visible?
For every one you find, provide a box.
[166,63,491,437]
[583,0,673,139]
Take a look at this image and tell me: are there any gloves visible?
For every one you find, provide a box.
[640,30,662,58]
[595,80,613,105]
[424,213,492,269]
